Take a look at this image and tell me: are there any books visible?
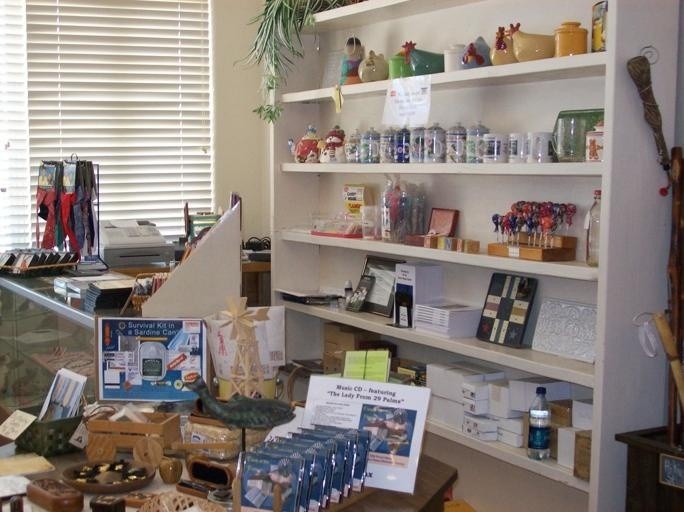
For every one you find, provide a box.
[413,298,484,340]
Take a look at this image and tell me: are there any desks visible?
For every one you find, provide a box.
[112,258,271,307]
[0,442,458,512]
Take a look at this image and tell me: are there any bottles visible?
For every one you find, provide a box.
[327,279,352,306]
[582,190,599,266]
[444,44,467,71]
[527,386,551,460]
[554,20,588,58]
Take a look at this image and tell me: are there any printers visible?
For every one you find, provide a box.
[98,219,174,267]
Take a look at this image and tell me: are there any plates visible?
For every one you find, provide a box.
[60,458,155,494]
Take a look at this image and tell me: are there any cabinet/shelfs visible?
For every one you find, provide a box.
[270,0,680,512]
[0,271,141,447]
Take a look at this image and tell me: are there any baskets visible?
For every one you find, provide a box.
[15,404,85,457]
[131,273,153,312]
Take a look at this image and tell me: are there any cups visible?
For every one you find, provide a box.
[358,184,429,241]
[284,107,603,164]
[389,57,411,78]
